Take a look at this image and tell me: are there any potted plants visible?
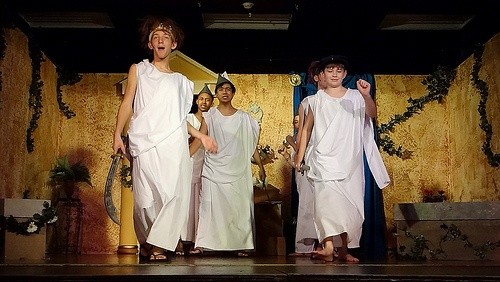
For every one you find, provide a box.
[49,155,92,198]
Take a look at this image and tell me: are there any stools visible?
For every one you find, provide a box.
[54,198,82,256]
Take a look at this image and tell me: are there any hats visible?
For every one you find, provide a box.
[201,85,211,95]
[216,73,229,87]
[320,47,351,70]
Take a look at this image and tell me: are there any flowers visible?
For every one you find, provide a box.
[26,216,58,233]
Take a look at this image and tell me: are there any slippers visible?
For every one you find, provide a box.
[234,251,249,257]
[189,247,214,256]
[150,252,167,261]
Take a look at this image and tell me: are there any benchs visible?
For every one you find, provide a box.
[3,198,52,264]
[393,201,500,261]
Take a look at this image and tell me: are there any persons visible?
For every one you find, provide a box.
[277,55,376,262]
[188,73,274,257]
[113,19,218,260]
